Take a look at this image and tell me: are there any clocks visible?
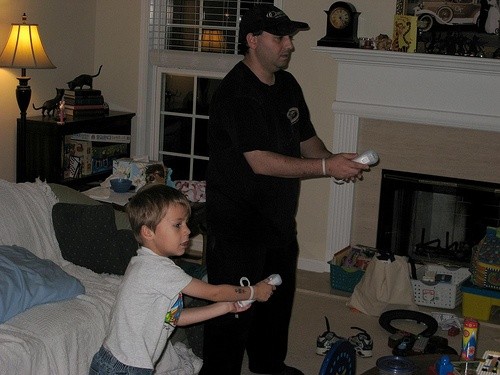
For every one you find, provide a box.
[318,2,362,48]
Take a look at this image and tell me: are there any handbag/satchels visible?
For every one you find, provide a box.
[345,250,420,317]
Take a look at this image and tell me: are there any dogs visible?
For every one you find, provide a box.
[146,164,165,184]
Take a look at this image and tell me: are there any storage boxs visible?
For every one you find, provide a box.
[17,108,136,192]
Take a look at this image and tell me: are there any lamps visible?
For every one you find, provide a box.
[1,10,55,117]
[194,27,230,54]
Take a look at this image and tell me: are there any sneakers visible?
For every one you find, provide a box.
[315,316,346,356]
[348,327,374,358]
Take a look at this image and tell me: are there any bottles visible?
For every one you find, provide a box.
[460,317,478,362]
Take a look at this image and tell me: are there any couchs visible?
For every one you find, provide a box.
[0,182,186,375]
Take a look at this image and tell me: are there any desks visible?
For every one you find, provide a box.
[361,355,500,375]
[85,167,206,218]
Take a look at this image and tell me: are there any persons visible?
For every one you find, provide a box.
[203,6,370,373]
[89,185,276,374]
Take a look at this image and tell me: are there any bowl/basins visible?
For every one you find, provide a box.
[110,179,131,193]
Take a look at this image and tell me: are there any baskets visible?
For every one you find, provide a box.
[409,262,473,309]
[327,260,365,293]
[472,230,500,291]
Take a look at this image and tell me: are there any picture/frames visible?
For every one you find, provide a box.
[396,0,488,57]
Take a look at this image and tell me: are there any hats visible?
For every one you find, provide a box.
[238,4,310,43]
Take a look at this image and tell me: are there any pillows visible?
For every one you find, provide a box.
[53,202,138,275]
[1,245,86,324]
